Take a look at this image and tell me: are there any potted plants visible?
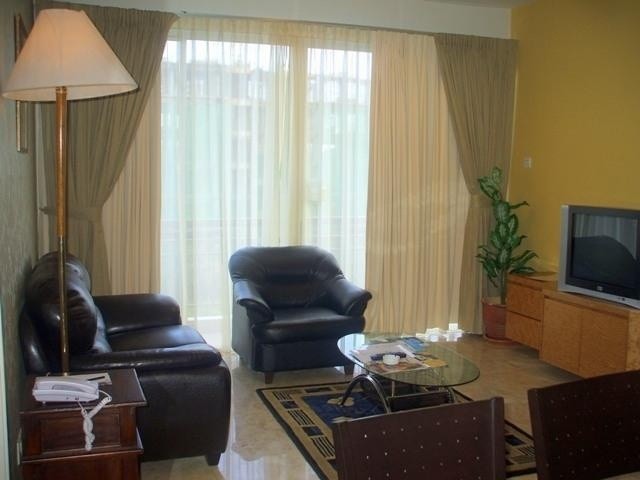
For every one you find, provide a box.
[477,166,541,344]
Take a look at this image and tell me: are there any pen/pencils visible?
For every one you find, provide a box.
[88,376,104,381]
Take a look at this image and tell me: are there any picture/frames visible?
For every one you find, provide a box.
[14,11,30,152]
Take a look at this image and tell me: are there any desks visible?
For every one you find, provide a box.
[20,366,147,480]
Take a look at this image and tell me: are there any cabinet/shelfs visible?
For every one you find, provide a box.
[541,288,640,378]
[505,268,557,349]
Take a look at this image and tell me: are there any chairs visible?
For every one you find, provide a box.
[332,395,505,480]
[528,370,639,480]
[229,245,373,383]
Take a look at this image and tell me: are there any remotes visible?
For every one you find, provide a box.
[370,351,407,360]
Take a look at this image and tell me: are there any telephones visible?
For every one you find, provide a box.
[32,376,102,406]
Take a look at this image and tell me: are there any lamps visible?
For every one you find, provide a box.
[2,10,139,374]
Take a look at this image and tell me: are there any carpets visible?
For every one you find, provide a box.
[257,383,535,478]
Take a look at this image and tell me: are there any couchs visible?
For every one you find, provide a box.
[24,252,232,466]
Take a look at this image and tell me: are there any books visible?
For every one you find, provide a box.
[35,371,113,386]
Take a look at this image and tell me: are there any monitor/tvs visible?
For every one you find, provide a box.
[557,204,640,307]
